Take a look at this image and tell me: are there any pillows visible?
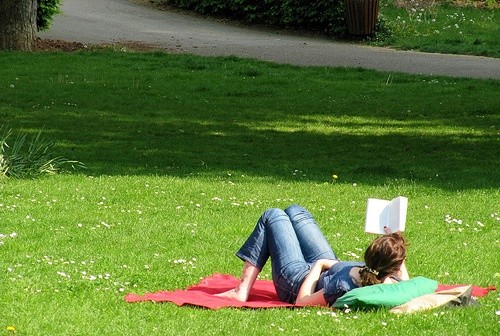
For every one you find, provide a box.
[389,284,472,316]
[332,275,439,309]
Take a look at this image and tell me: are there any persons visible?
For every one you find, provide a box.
[213,206,411,307]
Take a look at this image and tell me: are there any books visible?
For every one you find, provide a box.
[365,196,408,235]
